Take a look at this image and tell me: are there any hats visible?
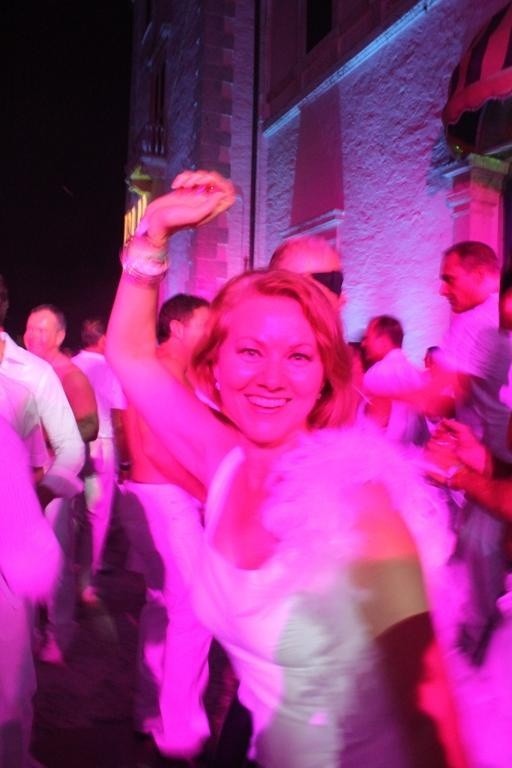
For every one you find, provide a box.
[268,234,344,276]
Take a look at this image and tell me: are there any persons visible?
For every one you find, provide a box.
[99,166,472,768]
[0,236,511,768]
